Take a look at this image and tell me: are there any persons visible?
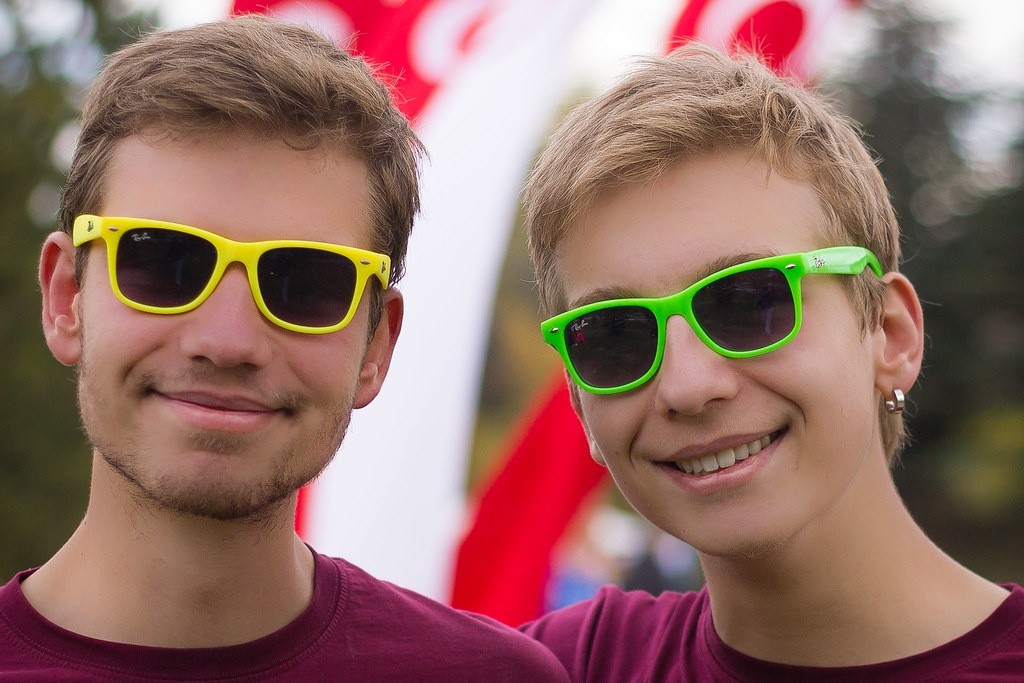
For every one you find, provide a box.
[509,44,1024,683]
[0,16,571,683]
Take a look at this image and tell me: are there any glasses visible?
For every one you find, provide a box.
[541,245,882,394]
[72,214,391,335]
